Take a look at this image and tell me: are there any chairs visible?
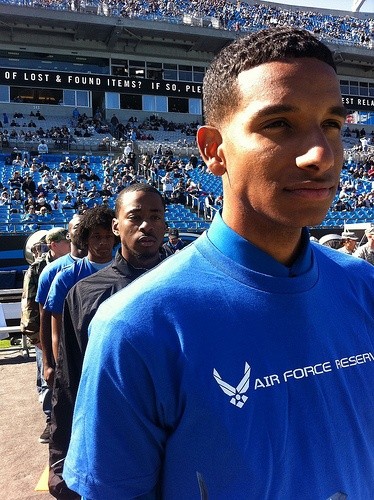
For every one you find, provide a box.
[0,0,374,232]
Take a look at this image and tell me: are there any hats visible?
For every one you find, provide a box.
[209,192,214,195]
[365,227,374,237]
[179,180,182,183]
[46,227,71,244]
[169,228,178,235]
[341,232,359,240]
[38,182,42,187]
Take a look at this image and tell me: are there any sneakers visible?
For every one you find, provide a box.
[39,422,51,443]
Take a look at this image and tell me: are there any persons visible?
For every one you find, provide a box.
[48,183,174,496]
[0,0,374,263]
[61,28,374,496]
[34,214,88,410]
[38,208,109,444]
